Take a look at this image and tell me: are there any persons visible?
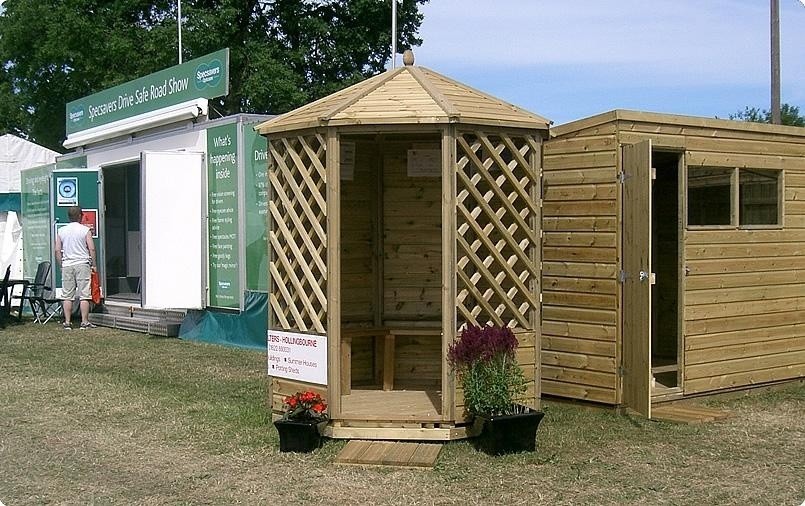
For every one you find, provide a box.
[55,205,98,331]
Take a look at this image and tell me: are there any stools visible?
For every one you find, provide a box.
[339,328,442,396]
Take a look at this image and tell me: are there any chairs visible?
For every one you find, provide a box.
[0,261,60,324]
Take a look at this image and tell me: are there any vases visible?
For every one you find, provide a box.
[468,405,545,456]
[274,419,329,453]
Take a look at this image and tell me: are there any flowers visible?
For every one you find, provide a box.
[281,390,329,422]
[445,323,528,418]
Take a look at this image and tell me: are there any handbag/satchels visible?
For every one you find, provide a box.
[91,271,102,305]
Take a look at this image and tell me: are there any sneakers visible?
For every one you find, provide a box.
[80,321,98,330]
[62,322,72,330]
